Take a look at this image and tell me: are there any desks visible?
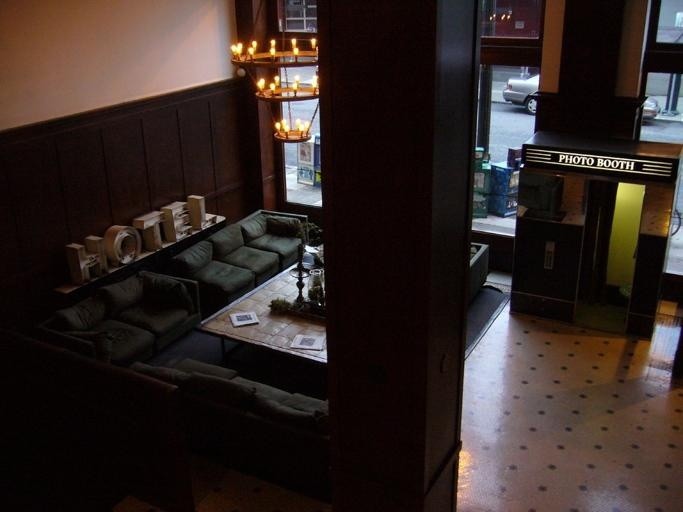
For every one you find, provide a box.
[195,263,327,372]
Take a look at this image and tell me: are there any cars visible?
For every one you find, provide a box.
[502,73,661,124]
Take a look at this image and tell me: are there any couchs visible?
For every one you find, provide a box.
[37,208,310,365]
[125,355,330,503]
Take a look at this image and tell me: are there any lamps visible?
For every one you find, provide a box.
[229,1,318,144]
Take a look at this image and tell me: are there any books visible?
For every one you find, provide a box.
[295,134,320,188]
[289,333,325,352]
[228,311,259,328]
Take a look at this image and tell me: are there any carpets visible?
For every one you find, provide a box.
[151,287,511,402]
